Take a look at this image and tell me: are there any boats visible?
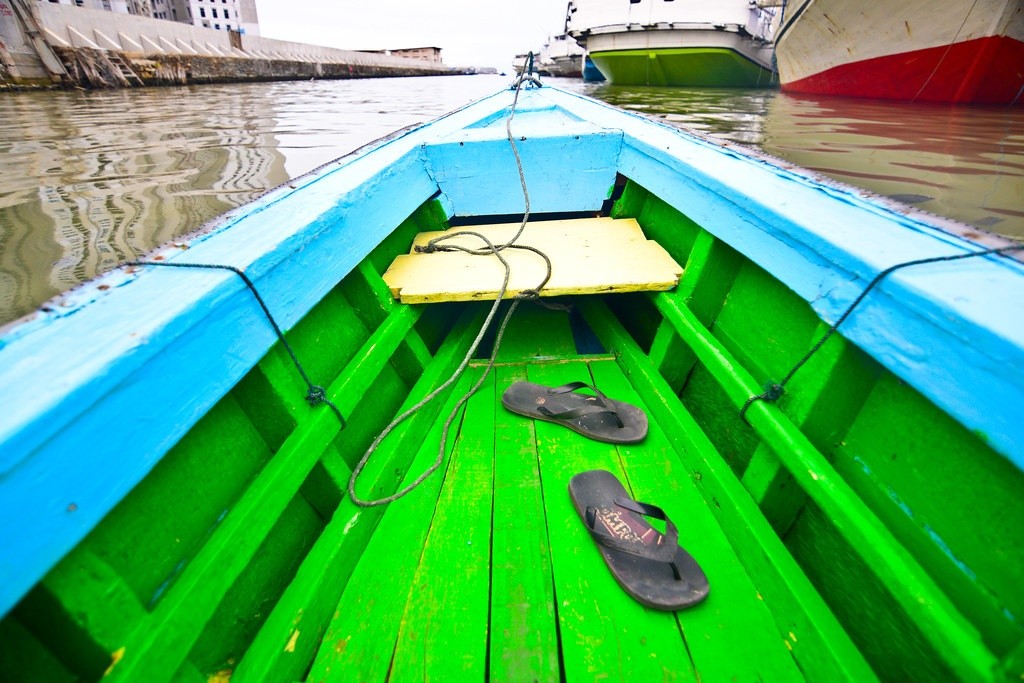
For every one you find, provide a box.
[466,66,478,74]
[511,33,605,81]
[500,72,505,75]
[565,0,777,89]
[756,0,1024,103]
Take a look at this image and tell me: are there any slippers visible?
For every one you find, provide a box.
[502,380,648,444]
[569,470,710,611]
